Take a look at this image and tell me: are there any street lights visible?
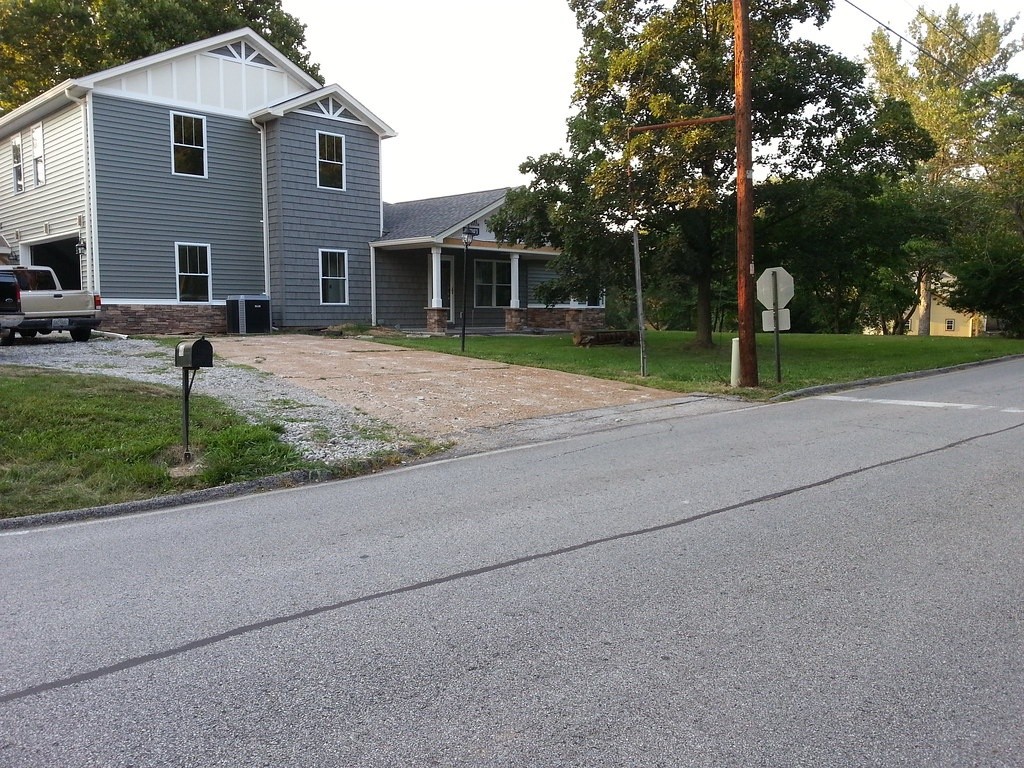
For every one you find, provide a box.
[460,225,480,353]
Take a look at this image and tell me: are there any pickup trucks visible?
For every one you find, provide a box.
[0,269,25,346]
[11,265,103,344]
